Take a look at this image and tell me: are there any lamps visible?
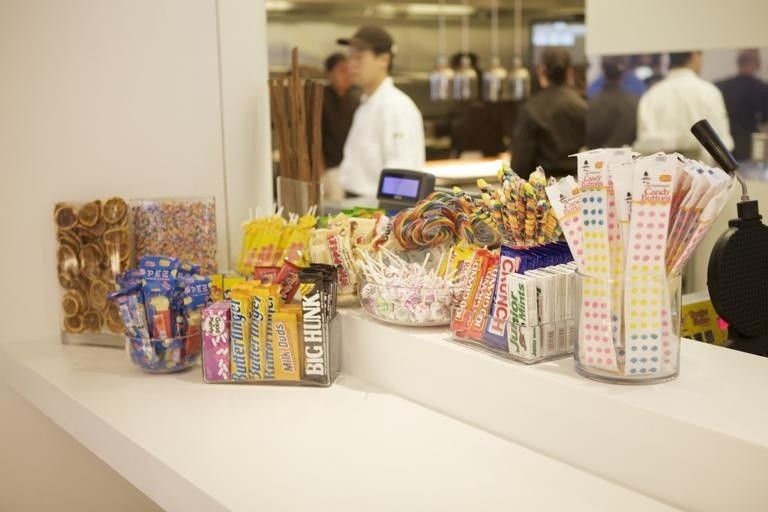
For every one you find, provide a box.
[427,1,531,105]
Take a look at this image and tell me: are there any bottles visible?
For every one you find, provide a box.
[429,53,531,102]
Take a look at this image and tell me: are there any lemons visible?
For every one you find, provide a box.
[54,197,132,334]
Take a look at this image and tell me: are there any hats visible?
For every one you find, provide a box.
[337,25,392,49]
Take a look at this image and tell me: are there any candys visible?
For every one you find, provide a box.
[236,163,564,323]
[130,202,217,275]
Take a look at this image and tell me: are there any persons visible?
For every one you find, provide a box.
[644,54,665,89]
[578,53,649,102]
[510,48,589,187]
[582,53,641,153]
[709,49,767,157]
[318,53,364,171]
[313,24,428,209]
[635,52,736,165]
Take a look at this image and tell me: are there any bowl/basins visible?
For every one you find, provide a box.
[121,328,203,375]
[356,273,470,328]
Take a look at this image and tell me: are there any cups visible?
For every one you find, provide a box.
[572,261,685,388]
